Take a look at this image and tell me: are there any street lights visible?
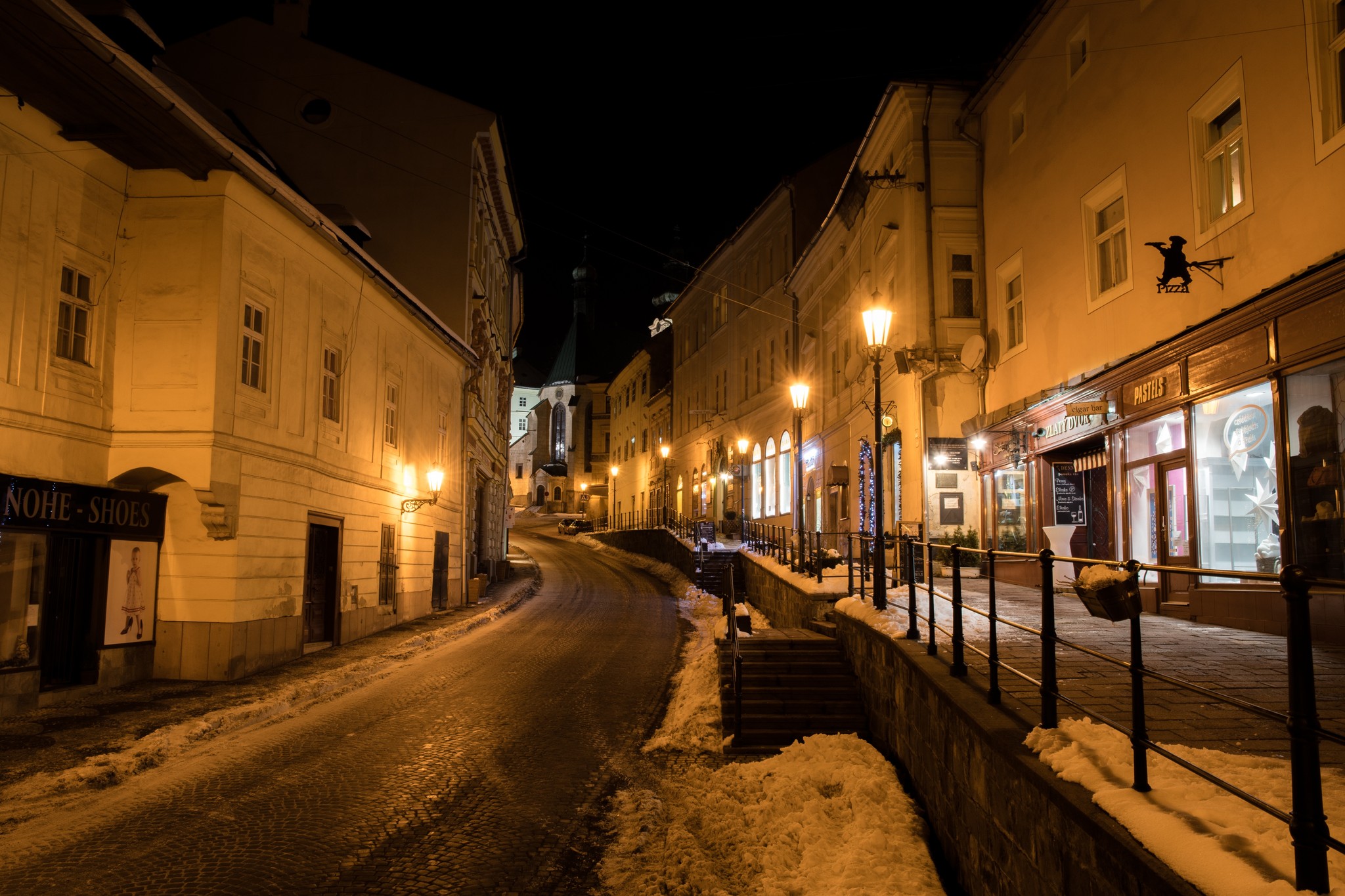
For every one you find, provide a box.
[736,438,749,543]
[788,379,812,572]
[858,288,895,611]
[580,481,587,520]
[611,466,618,528]
[661,446,669,528]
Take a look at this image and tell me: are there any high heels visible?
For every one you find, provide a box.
[137,619,143,640]
[120,616,133,634]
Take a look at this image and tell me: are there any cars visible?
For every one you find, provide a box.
[567,520,594,536]
[558,519,576,534]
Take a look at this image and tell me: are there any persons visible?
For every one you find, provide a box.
[120,546,146,640]
